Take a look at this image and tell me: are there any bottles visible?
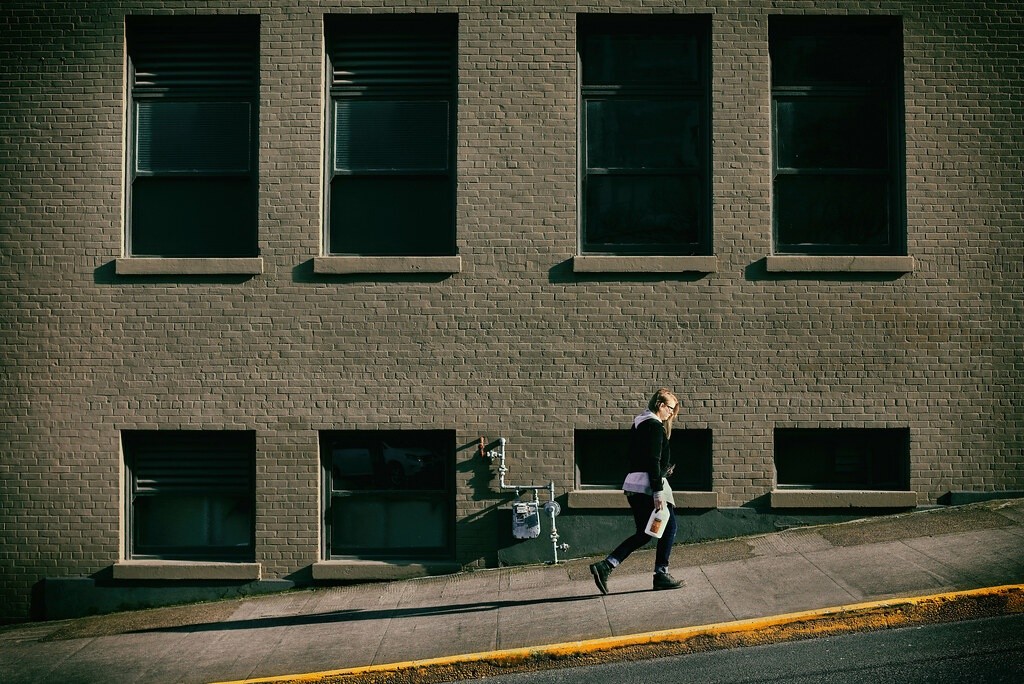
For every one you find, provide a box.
[644,502,670,538]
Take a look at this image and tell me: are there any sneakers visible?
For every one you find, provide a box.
[653,573,685,590]
[590,561,609,594]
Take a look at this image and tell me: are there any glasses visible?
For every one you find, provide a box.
[667,406,674,410]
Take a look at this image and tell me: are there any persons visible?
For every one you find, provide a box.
[590,388,687,594]
